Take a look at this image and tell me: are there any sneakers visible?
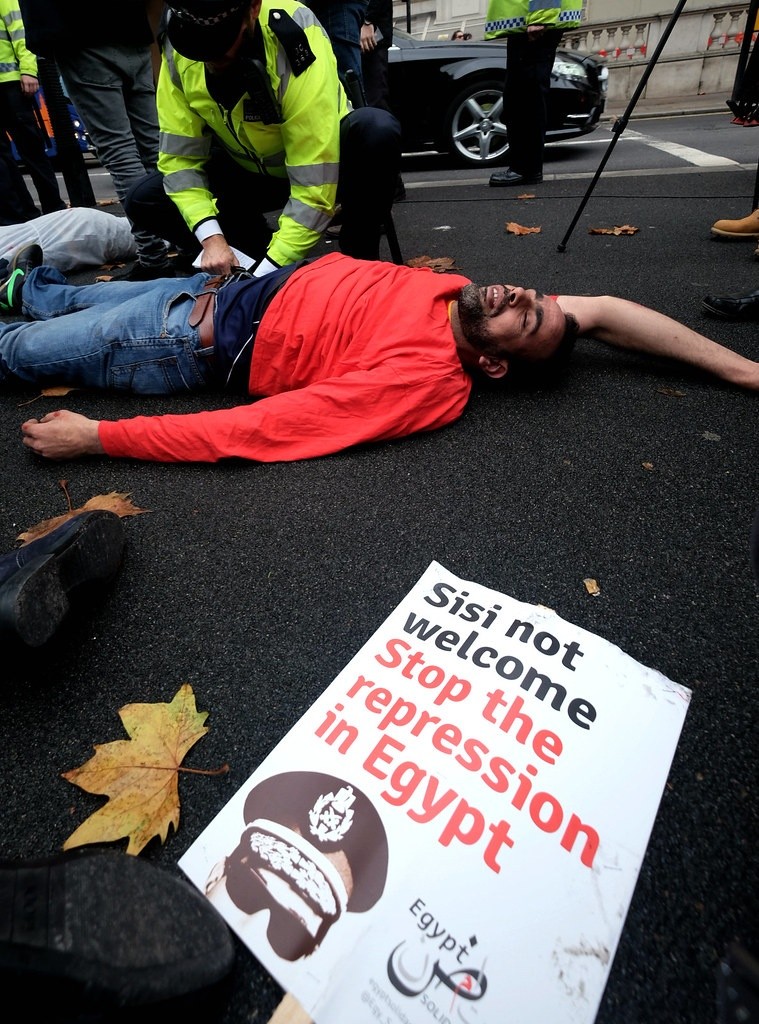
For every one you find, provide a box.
[0,244,43,315]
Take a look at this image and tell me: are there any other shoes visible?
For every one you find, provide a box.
[701,291,759,320]
[710,209,759,237]
[0,851,235,1003]
[111,265,176,281]
[0,510,125,650]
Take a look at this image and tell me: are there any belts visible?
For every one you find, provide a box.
[190,273,233,365]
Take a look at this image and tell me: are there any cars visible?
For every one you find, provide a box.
[388,25,610,169]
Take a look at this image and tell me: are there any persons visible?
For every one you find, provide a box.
[451,30,465,41]
[712,209,759,255]
[0,0,404,278]
[1,244,759,463]
[483,0,584,188]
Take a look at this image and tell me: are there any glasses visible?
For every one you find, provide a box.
[456,36,464,39]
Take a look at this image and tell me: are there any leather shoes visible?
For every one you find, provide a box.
[489,171,543,186]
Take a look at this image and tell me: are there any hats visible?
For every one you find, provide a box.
[167,0,252,62]
